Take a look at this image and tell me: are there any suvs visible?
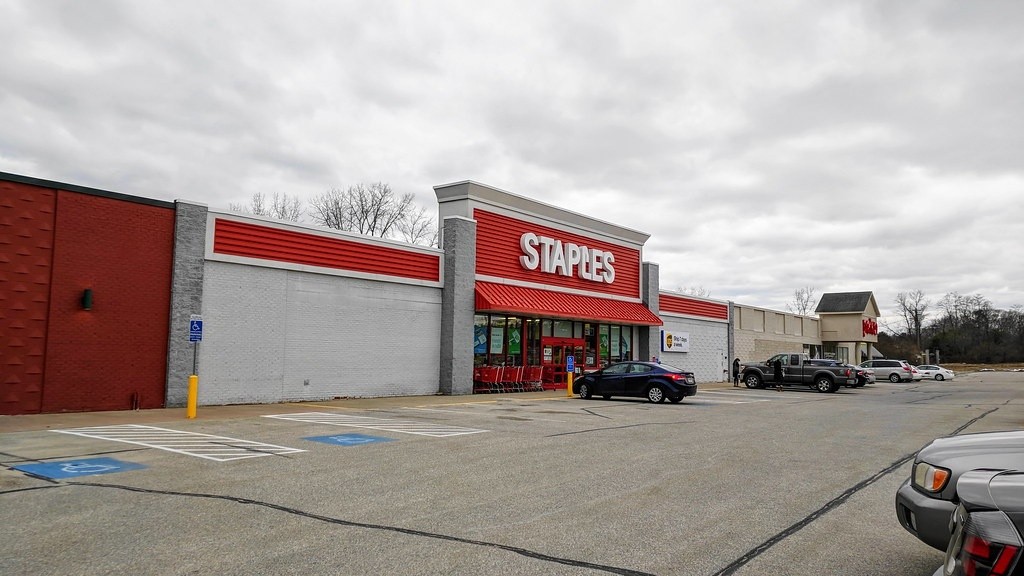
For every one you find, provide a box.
[805,359,844,390]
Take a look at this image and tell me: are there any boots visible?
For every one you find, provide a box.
[776,387,780,392]
[781,387,783,391]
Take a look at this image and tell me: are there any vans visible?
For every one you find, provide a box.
[857,360,913,383]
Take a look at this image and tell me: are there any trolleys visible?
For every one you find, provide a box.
[472,363,545,394]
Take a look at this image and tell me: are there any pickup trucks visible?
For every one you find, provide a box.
[737,352,859,393]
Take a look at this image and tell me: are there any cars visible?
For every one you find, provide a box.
[934,468,1024,576]
[846,364,876,387]
[916,365,955,381]
[894,429,1024,554]
[572,360,697,404]
[909,365,923,381]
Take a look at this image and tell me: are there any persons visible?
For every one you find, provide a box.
[733,358,740,387]
[774,359,783,392]
